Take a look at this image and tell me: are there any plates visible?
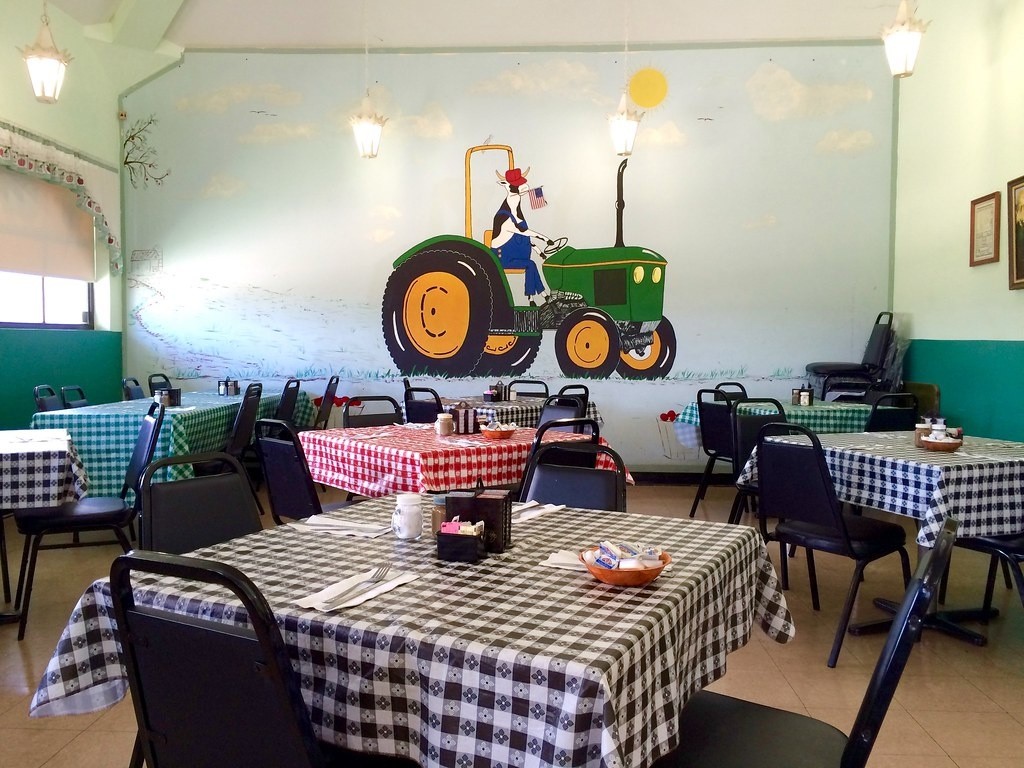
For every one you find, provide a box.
[434,419,455,433]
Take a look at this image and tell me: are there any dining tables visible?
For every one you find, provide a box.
[29,493,795,768]
[0,429,90,624]
[31,391,314,512]
[297,395,635,498]
[735,432,1024,646]
[673,401,872,446]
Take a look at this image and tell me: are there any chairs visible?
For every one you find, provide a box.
[109,549,327,767]
[139,451,263,555]
[650,517,959,768]
[690,382,913,667]
[196,376,340,475]
[343,378,443,501]
[15,401,165,640]
[508,380,627,512]
[255,418,366,524]
[805,313,906,402]
[34,373,171,411]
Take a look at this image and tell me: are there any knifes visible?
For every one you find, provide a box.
[322,571,405,610]
[311,528,379,533]
[511,507,545,515]
[551,563,588,568]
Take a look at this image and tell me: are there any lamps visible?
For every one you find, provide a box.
[15,0,75,103]
[347,0,390,158]
[879,0,932,78]
[606,0,644,156]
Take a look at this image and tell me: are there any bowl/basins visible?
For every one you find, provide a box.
[579,547,672,586]
[921,439,962,452]
[479,429,516,439]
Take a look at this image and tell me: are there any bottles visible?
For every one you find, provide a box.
[957,429,963,446]
[915,424,930,448]
[154,391,161,406]
[443,405,457,413]
[800,392,809,406]
[792,390,799,405]
[932,425,946,439]
[510,390,516,400]
[392,494,423,541]
[228,382,234,396]
[476,406,497,423]
[437,414,453,435]
[432,495,446,539]
[161,391,169,407]
[219,383,226,396]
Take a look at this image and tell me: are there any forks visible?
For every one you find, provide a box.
[300,523,391,533]
[511,504,543,519]
[322,563,392,603]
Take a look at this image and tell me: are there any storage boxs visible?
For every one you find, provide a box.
[435,530,487,562]
[483,394,500,402]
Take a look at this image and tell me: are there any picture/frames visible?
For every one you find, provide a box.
[970,191,1001,266]
[1008,176,1024,289]
